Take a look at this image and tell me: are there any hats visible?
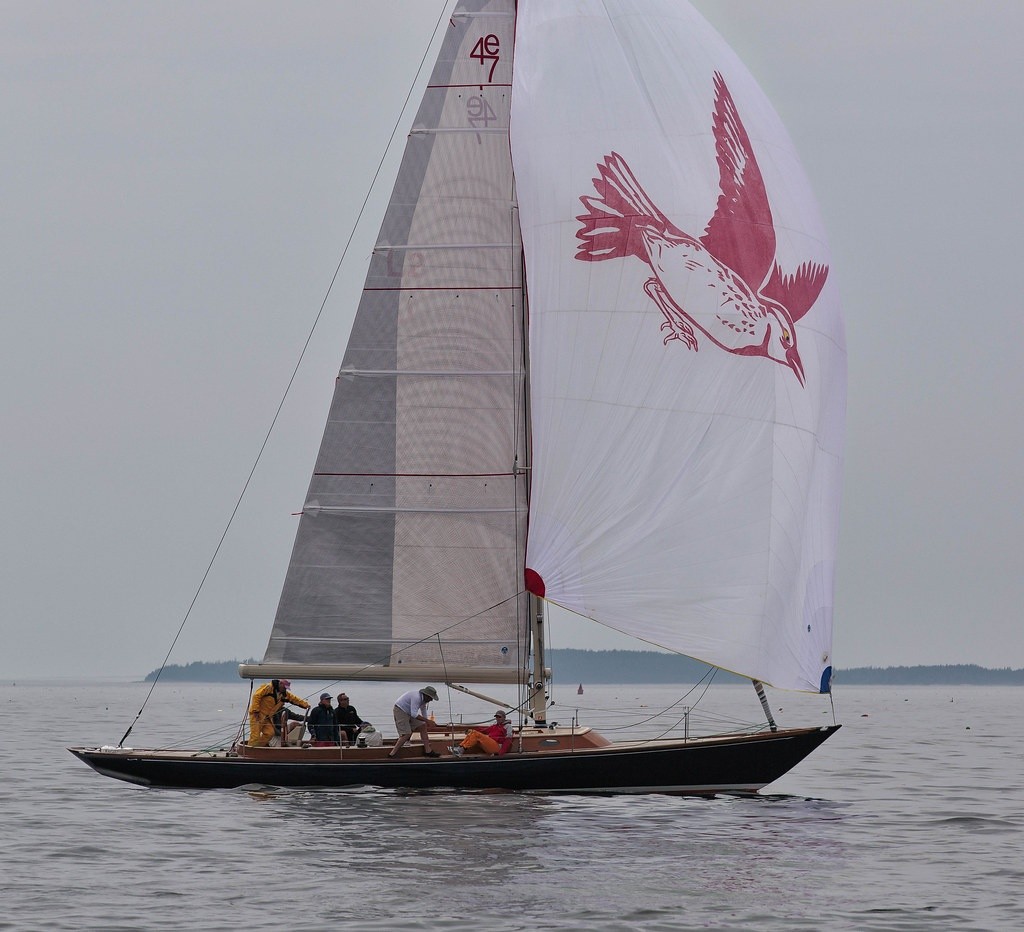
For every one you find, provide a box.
[320,693,333,700]
[419,686,439,701]
[281,679,291,690]
[494,710,506,719]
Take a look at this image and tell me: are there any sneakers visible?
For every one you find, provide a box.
[451,747,462,758]
[447,745,454,752]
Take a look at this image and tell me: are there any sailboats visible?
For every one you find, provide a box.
[71,0,851,795]
[578,684,584,693]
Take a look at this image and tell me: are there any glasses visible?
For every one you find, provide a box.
[325,697,331,700]
[495,715,502,718]
[344,697,349,701]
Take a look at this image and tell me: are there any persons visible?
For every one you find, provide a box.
[274,707,308,747]
[248,679,310,747]
[387,686,441,759]
[355,722,383,747]
[333,693,363,746]
[307,693,338,741]
[446,710,513,757]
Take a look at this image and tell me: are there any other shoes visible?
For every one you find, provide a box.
[388,752,402,759]
[426,749,442,758]
